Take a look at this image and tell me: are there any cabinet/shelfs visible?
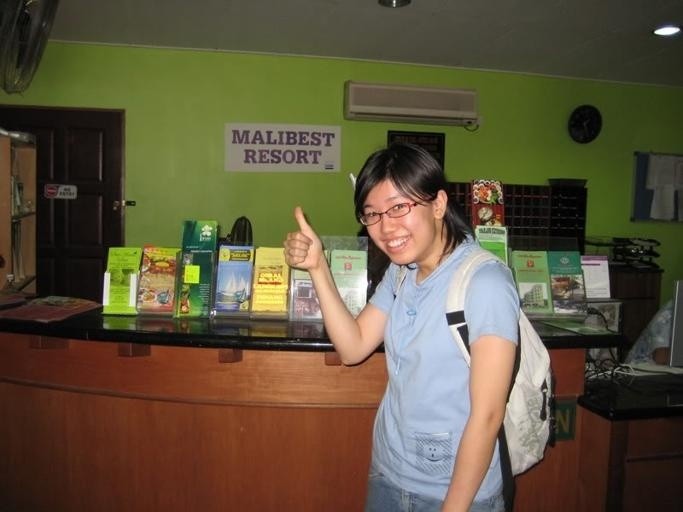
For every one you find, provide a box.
[608,272,662,350]
[446,177,588,256]
[1,135,37,297]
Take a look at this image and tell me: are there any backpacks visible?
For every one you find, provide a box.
[392,248,552,512]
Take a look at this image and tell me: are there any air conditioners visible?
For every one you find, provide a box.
[344,79,478,126]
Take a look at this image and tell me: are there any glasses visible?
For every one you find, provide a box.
[358,195,430,226]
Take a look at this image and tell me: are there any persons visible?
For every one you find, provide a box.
[284,143,520,512]
[624,297,675,365]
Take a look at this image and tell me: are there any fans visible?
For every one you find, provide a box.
[0,0,60,94]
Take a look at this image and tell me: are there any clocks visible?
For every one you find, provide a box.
[568,105,602,144]
